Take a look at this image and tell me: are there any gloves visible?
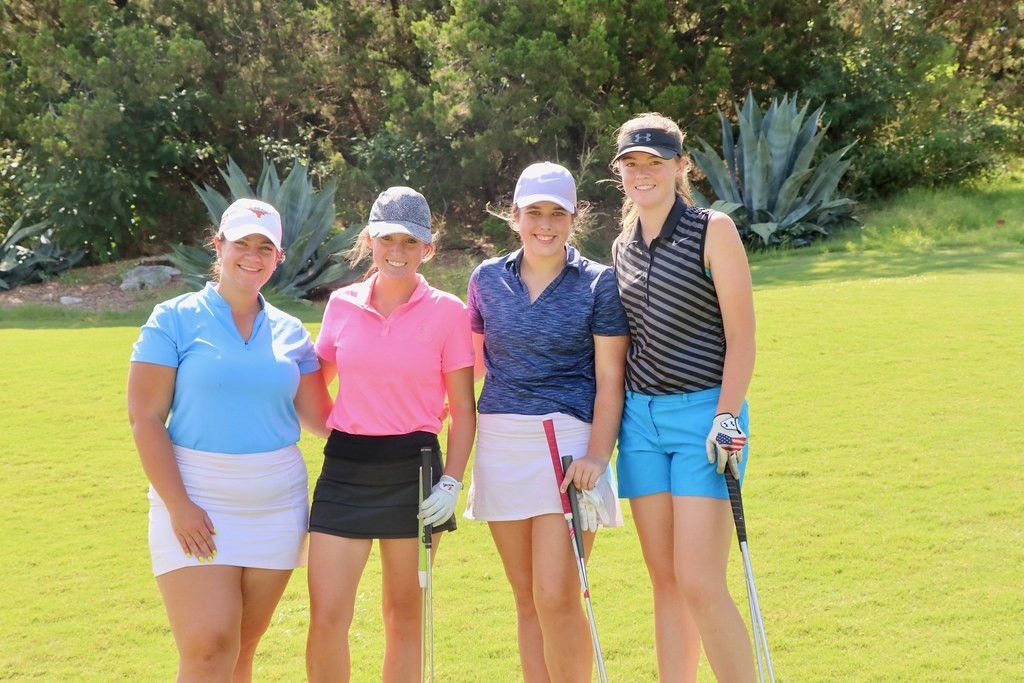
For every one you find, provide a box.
[706,412,747,480]
[578,480,610,532]
[417,475,462,527]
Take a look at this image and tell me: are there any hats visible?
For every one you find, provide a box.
[612,129,683,164]
[513,160,578,214]
[218,198,282,253]
[368,184,433,244]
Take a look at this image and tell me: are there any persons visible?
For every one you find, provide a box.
[467,159,631,683]
[612,114,756,683]
[306,186,476,683]
[128,197,449,683]
